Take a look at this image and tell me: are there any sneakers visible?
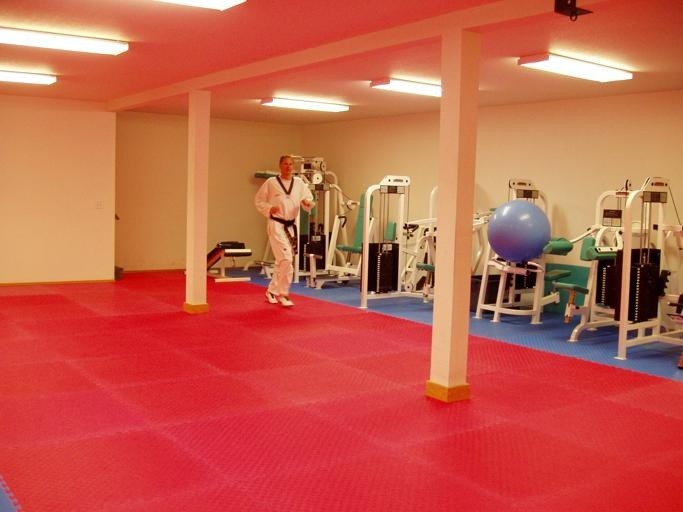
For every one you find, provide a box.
[267,291,278,304]
[279,295,294,306]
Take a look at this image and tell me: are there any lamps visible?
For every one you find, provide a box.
[0,70,57,85]
[517,53,633,83]
[259,97,350,112]
[370,78,442,98]
[0,24,129,56]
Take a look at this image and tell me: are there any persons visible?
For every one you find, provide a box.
[254,155,315,306]
